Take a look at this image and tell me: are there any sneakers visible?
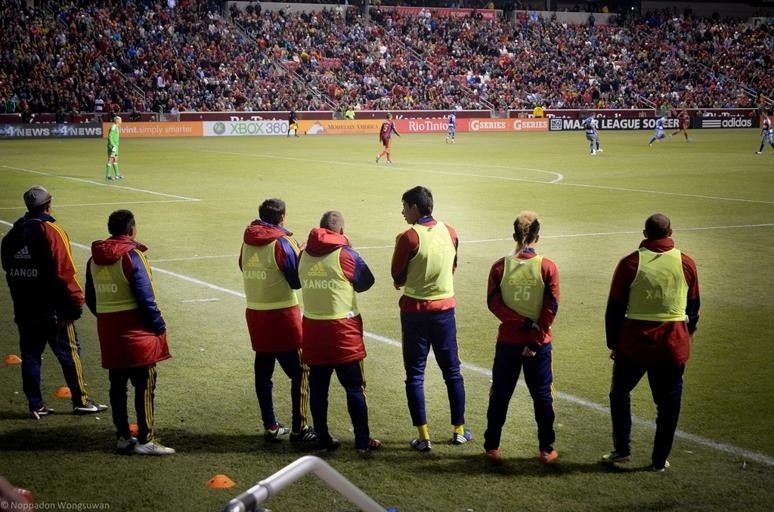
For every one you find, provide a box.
[446,137,455,143]
[755,151,762,155]
[591,148,602,155]
[263,421,289,438]
[412,437,431,451]
[356,440,381,452]
[289,424,340,450]
[452,429,473,443]
[487,448,500,461]
[108,176,124,181]
[603,450,632,463]
[74,400,107,414]
[651,459,670,472]
[114,437,176,454]
[376,155,393,164]
[31,406,54,418]
[541,449,558,463]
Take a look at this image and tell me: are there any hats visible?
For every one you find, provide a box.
[23,185,51,211]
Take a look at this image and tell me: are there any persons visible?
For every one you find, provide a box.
[754,110,774,156]
[483,209,560,465]
[390,184,474,452]
[2,2,774,119]
[445,110,458,145]
[1,185,110,421]
[297,209,382,454]
[647,112,669,149]
[602,212,701,474]
[84,208,180,457]
[669,106,694,144]
[584,111,604,156]
[104,115,126,181]
[375,112,402,165]
[287,106,300,138]
[237,196,318,445]
